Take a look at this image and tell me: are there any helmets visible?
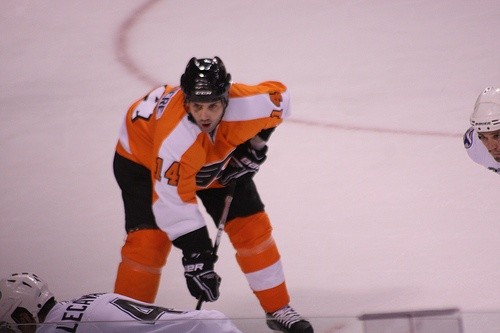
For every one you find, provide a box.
[181,56,232,102]
[0,272,54,333]
[471,86,500,132]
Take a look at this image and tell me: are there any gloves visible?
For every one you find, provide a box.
[182,249,220,302]
[217,139,268,190]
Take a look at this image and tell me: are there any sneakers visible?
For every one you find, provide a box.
[266,305,314,333]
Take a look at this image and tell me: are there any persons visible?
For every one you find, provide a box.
[0,272,245,333]
[463,84,500,174]
[113,56,315,333]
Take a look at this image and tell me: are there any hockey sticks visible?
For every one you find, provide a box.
[194,181,237,310]
